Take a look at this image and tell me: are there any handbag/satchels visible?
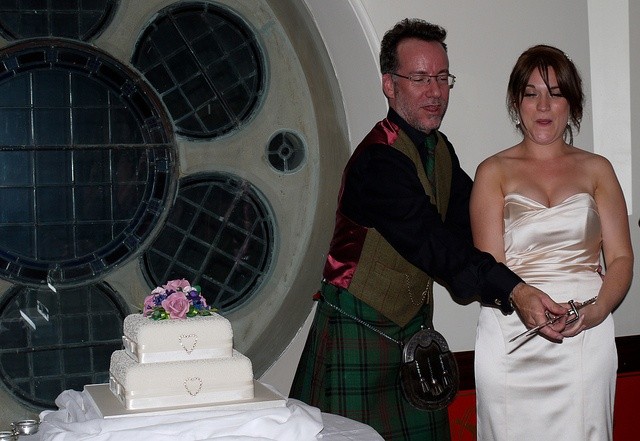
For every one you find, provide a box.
[317,295,460,412]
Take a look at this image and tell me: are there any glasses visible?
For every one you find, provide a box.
[384,70,455,90]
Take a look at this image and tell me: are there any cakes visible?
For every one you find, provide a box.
[108,279,254,412]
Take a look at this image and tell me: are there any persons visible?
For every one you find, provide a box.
[469,44,635,439]
[287,17,570,440]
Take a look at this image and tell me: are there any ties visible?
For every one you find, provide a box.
[424,136,437,194]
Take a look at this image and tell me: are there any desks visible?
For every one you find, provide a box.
[8,402,384,441]
